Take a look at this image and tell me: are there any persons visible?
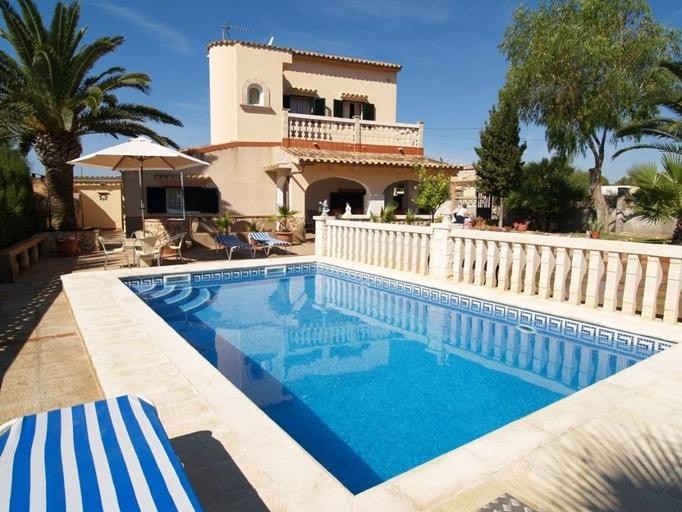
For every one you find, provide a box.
[452,203,469,224]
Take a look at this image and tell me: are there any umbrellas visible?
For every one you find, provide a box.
[65,135,212,239]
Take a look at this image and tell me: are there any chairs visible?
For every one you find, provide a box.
[248,232,291,256]
[215,235,256,260]
[97,230,187,271]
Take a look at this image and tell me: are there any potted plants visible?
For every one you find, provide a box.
[582,215,604,238]
[276,207,299,242]
